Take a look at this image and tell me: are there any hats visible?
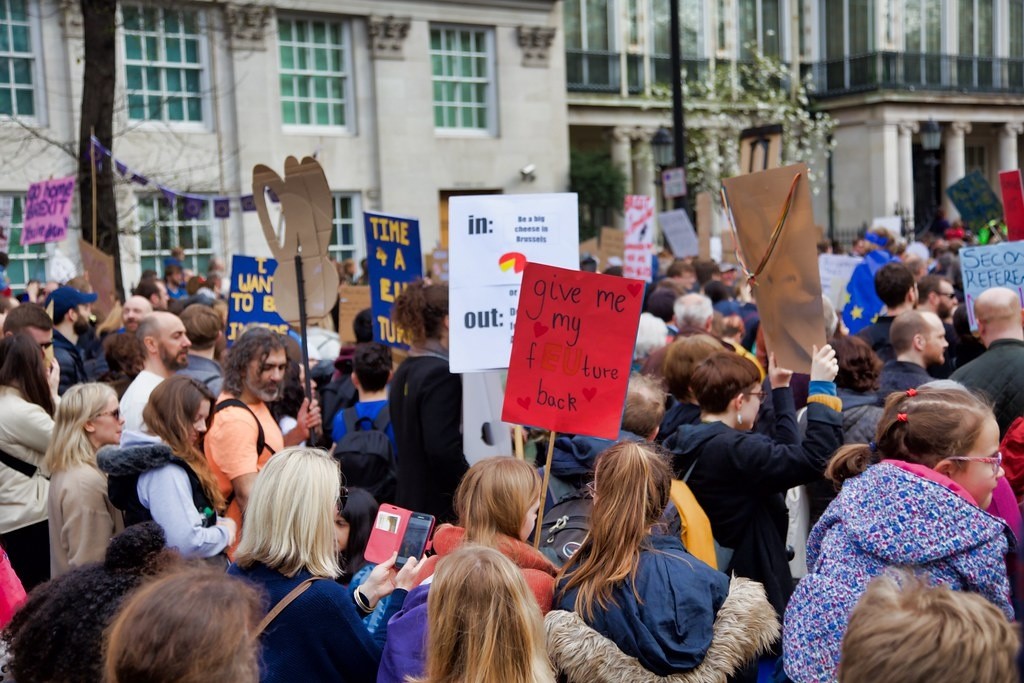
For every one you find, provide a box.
[3,522,169,683]
[45,285,98,318]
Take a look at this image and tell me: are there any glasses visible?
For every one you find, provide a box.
[945,451,1002,474]
[936,292,956,299]
[334,486,349,509]
[96,408,120,421]
[586,481,599,499]
[746,391,769,405]
[39,341,54,349]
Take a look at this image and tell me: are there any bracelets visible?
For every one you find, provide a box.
[354,585,376,614]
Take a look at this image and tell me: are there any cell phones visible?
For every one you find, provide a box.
[395,512,434,570]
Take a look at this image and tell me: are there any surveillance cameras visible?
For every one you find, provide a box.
[520,163,536,176]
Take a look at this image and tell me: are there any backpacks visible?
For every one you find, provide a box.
[332,402,398,506]
[316,373,359,449]
[533,468,606,570]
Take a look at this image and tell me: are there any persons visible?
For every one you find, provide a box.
[0,222,1024,683]
